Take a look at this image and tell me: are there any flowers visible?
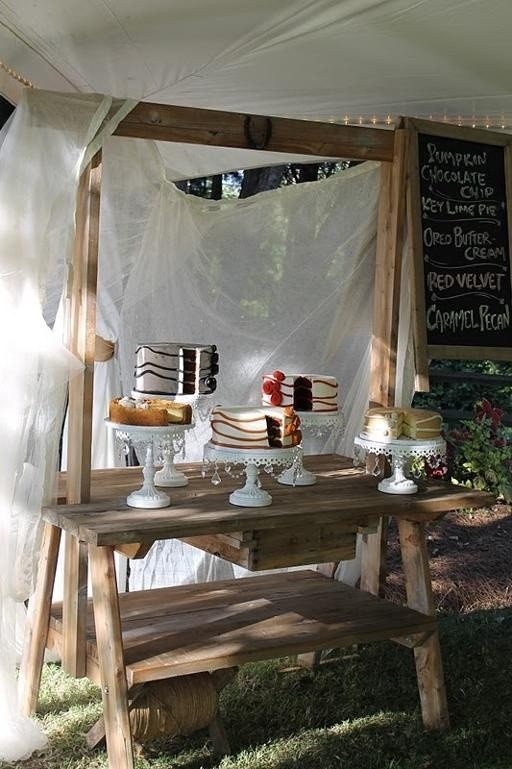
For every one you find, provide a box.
[439,397,512,475]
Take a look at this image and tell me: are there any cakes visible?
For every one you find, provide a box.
[133,343,219,394]
[108,396,192,427]
[261,370,338,413]
[212,405,301,448]
[363,407,444,438]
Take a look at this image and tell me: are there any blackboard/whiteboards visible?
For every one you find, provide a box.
[396,114,512,363]
